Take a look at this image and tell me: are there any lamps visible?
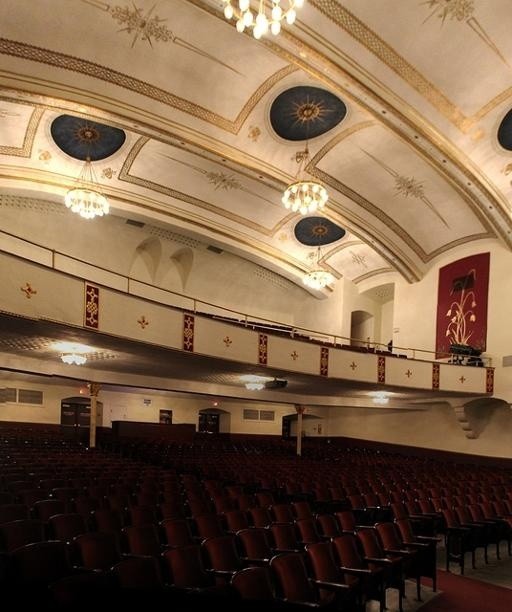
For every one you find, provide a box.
[64,130,111,221]
[222,0,308,39]
[302,230,334,291]
[281,107,329,214]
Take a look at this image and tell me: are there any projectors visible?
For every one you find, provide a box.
[264,380,287,390]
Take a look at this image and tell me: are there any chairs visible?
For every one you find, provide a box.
[0,420,512,612]
